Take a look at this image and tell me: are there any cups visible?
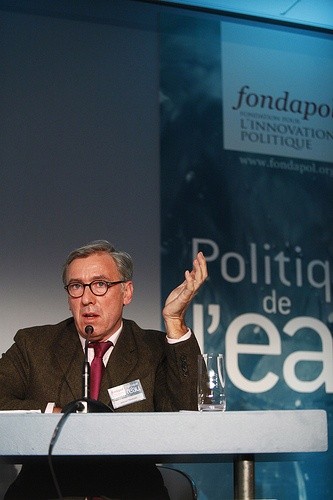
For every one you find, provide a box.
[197,353,226,413]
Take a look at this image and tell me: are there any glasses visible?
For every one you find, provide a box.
[63,279,127,298]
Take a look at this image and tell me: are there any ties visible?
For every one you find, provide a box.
[86,339,113,402]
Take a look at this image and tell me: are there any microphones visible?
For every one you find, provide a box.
[60,325,114,413]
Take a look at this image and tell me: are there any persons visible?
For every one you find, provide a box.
[0,238,214,500]
